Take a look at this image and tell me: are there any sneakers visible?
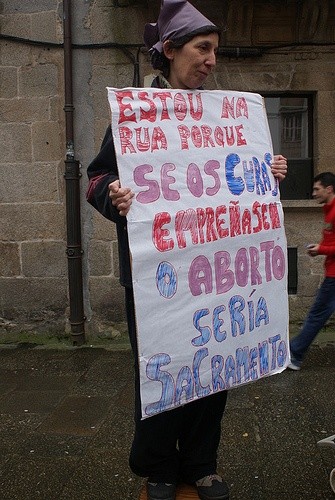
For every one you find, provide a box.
[146,480,180,500]
[287,349,305,372]
[197,473,230,499]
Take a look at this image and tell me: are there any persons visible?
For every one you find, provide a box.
[86,0,287,500]
[286,173,335,371]
